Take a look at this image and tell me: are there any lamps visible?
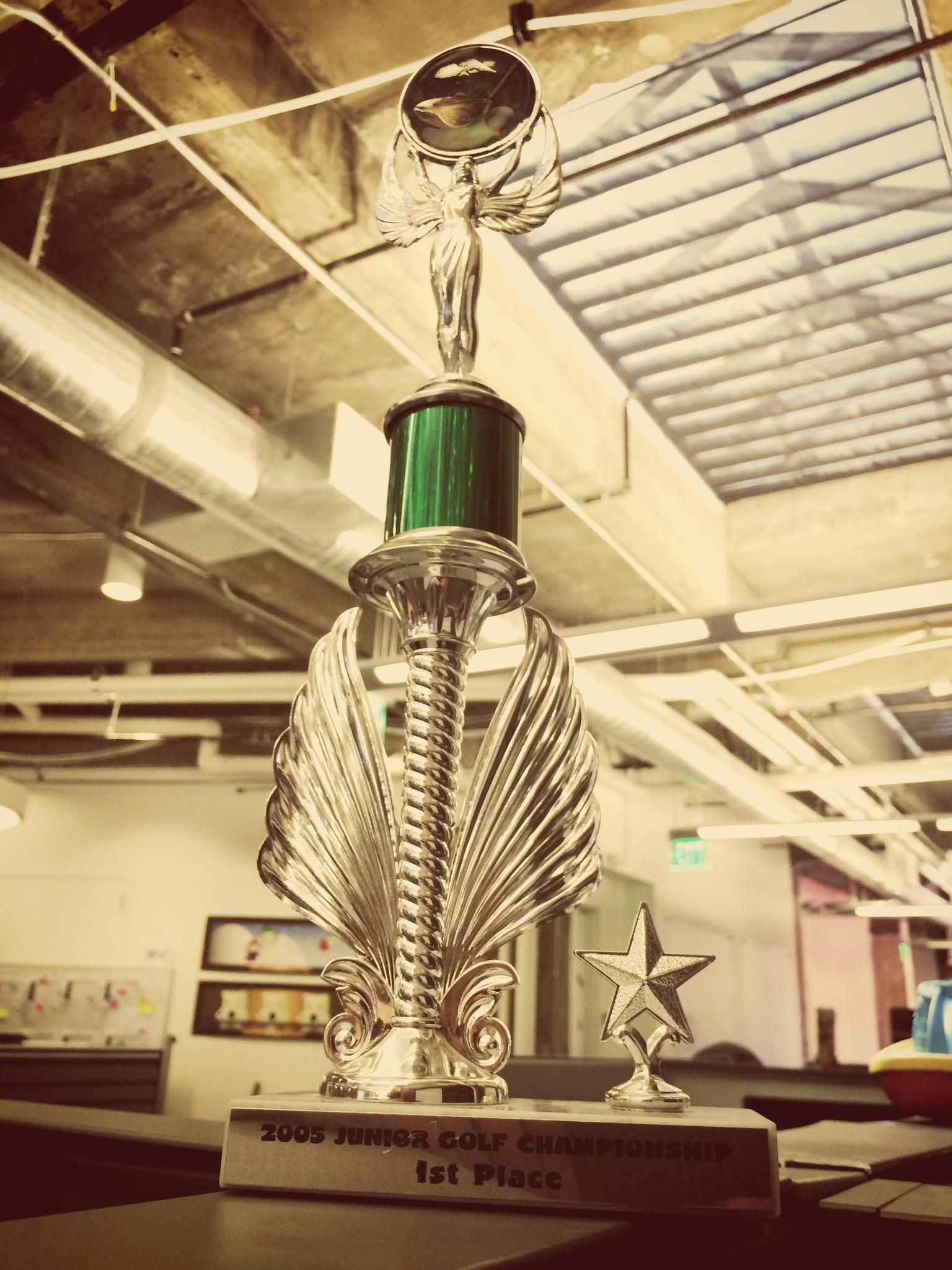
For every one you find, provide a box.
[100,471,147,602]
